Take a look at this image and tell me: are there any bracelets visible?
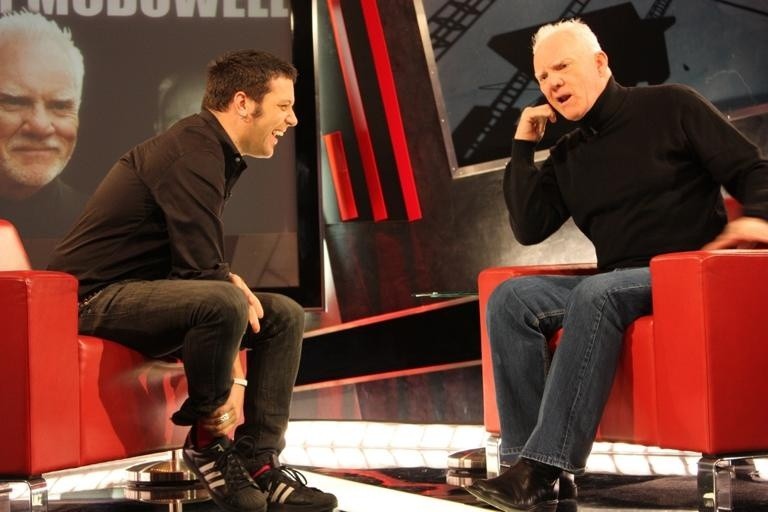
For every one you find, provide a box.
[230,376,250,388]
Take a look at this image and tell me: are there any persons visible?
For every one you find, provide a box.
[149,64,214,142]
[457,17,768,511]
[1,4,100,243]
[36,48,342,511]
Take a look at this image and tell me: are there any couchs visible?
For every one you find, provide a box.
[0,271,254,512]
[443,194,766,511]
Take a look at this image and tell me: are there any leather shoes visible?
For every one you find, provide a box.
[464,458,578,512]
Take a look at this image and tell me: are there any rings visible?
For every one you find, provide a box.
[214,414,229,424]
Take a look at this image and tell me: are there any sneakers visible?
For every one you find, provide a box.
[236,438,339,511]
[182,435,267,511]
